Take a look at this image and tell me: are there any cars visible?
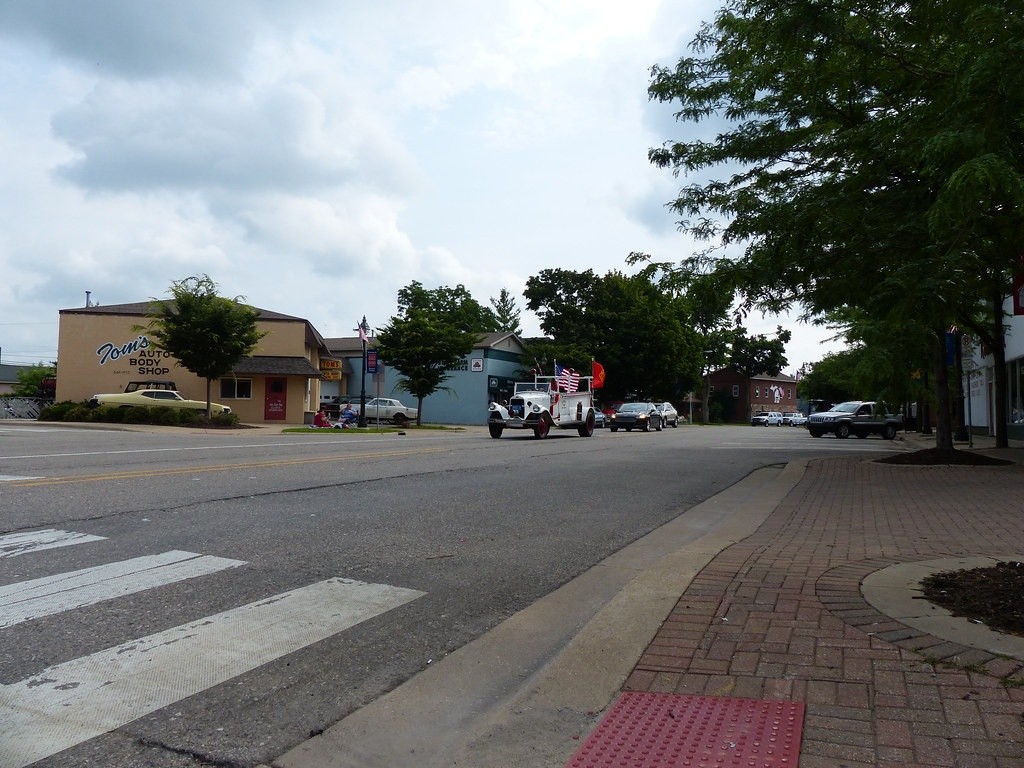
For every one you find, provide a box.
[610,402,664,432]
[588,406,607,429]
[339,397,419,423]
[488,357,605,440]
[92,388,232,417]
[648,401,678,428]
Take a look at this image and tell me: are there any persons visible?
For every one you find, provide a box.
[314,408,335,428]
[336,402,358,426]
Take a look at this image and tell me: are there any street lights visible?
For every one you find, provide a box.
[352,314,370,424]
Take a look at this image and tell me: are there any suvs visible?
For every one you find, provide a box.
[752,411,811,427]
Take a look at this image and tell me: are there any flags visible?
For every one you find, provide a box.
[796,371,801,380]
[769,383,778,393]
[589,361,605,388]
[554,365,581,392]
[357,325,370,345]
[778,385,785,397]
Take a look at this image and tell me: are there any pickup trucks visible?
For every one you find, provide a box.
[805,399,905,439]
